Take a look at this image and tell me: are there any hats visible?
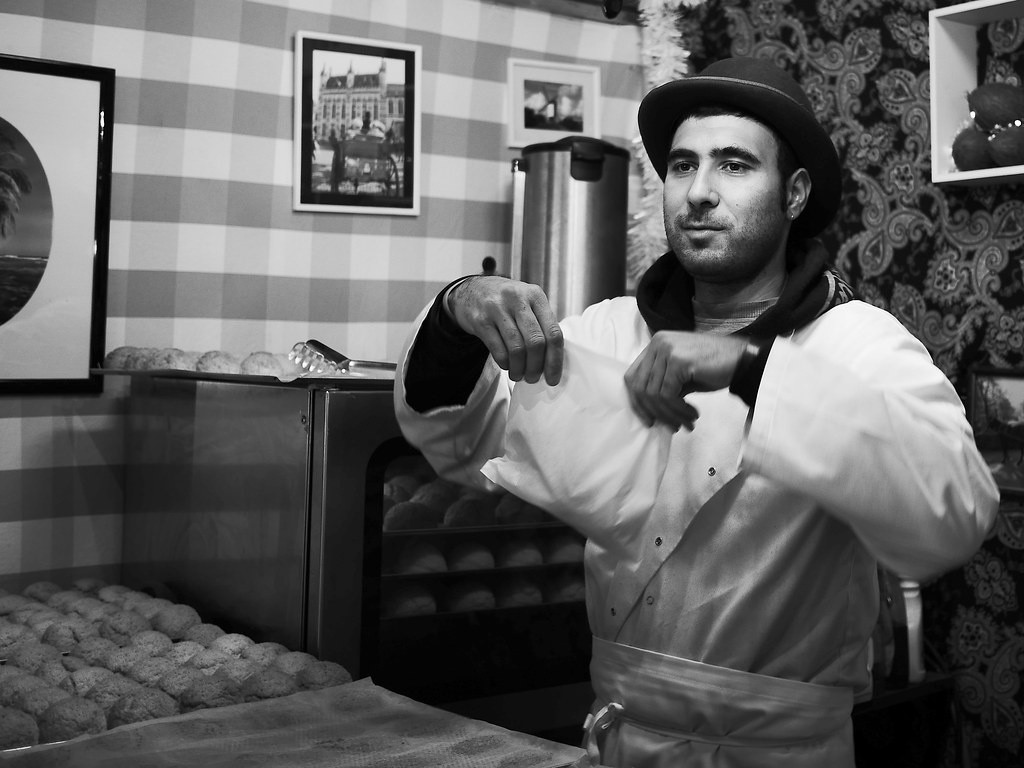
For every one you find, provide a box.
[637,55,844,239]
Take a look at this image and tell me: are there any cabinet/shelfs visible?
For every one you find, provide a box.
[928,0,1024,182]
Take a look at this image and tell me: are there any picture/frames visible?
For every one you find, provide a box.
[0,51,114,397]
[507,57,601,147]
[294,28,421,217]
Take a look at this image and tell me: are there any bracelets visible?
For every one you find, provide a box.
[728,336,765,395]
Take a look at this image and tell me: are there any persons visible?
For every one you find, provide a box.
[393,56,1001,768]
